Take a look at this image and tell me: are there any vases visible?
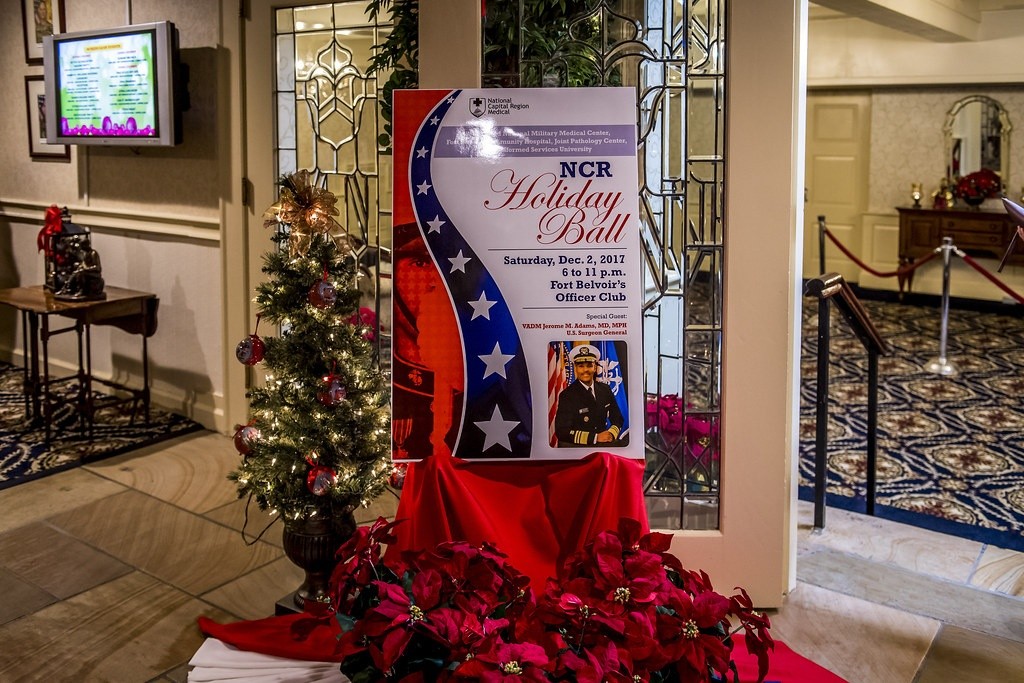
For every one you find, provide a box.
[962,193,984,211]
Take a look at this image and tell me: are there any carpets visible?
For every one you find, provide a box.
[0,360,204,492]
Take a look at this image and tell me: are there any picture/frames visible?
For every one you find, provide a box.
[24,75,71,160]
[21,0,66,63]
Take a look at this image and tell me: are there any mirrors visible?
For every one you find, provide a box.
[944,95,1012,206]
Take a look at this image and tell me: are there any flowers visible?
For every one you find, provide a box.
[646,395,716,480]
[952,171,997,202]
[325,515,773,683]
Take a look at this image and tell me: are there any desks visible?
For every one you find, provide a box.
[0,284,158,447]
[894,205,1024,300]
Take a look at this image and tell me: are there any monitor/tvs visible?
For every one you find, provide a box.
[42,20,183,148]
